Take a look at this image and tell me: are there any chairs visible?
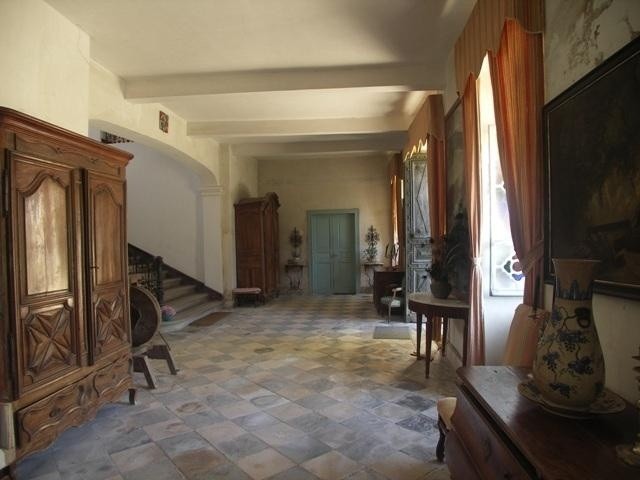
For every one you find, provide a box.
[380,276,406,324]
[437,304,549,462]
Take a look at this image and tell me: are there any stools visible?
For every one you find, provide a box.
[233,288,265,305]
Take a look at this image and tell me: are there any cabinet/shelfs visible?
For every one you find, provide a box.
[0,107,136,480]
[233,192,280,304]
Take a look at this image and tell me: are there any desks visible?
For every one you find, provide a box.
[444,366,640,480]
[284,264,306,294]
[363,263,383,293]
[374,266,405,317]
[408,290,469,378]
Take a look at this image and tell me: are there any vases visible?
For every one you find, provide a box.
[533,258,605,406]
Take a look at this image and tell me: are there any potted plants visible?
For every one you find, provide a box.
[423,202,471,299]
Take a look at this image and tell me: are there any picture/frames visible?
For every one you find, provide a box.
[543,33,640,301]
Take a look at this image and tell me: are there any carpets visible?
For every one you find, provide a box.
[373,326,410,339]
